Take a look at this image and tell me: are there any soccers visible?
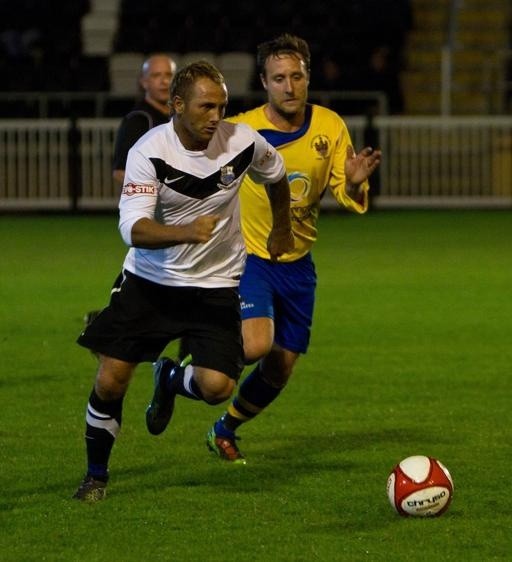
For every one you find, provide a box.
[387,455,453,517]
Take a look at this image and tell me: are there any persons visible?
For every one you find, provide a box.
[72,59,295,505]
[321,48,401,114]
[111,53,177,186]
[174,32,382,463]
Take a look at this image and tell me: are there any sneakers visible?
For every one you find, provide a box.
[145,357,174,435]
[206,422,248,466]
[72,475,107,504]
